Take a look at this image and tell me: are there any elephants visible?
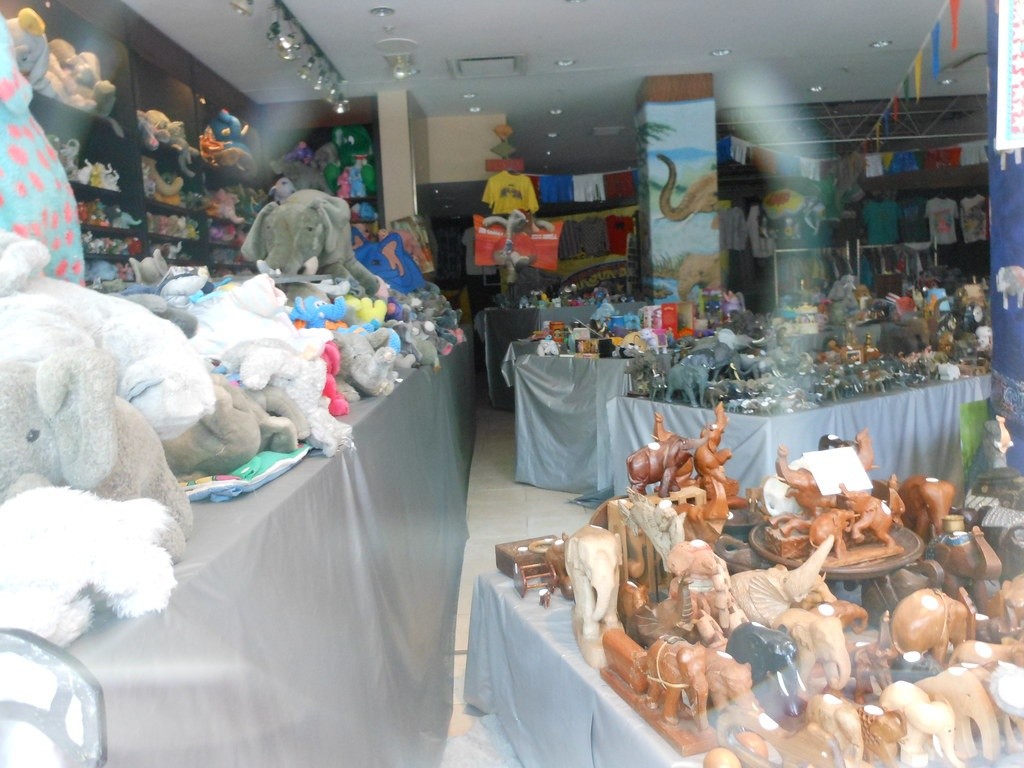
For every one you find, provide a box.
[566,330,1024,768]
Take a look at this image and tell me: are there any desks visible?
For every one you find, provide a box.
[606,373,992,496]
[500,340,634,494]
[474,300,648,413]
[65,324,478,768]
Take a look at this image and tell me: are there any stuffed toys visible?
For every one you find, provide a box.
[0,0,468,650]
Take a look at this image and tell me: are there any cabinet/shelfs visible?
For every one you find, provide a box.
[0,0,263,261]
[261,95,384,231]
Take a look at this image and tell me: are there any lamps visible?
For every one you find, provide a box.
[229,0,254,17]
[266,4,296,49]
[279,18,300,59]
[311,69,327,91]
[323,84,338,104]
[332,92,351,115]
[390,52,408,79]
[295,56,315,82]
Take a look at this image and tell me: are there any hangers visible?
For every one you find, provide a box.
[872,187,980,202]
[506,166,522,175]
